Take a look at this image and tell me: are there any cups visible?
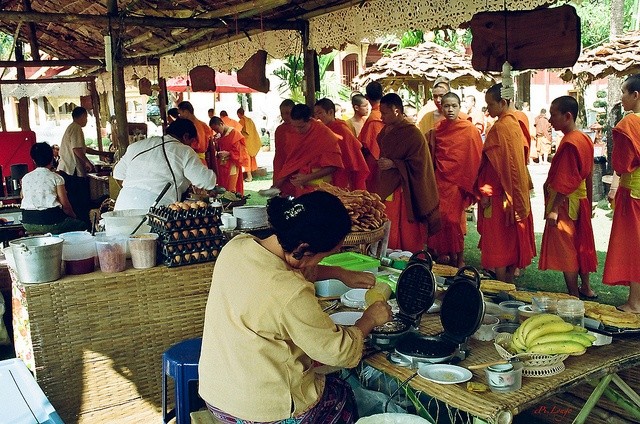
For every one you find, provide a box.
[223,215,237,231]
[518,304,538,324]
[488,364,514,391]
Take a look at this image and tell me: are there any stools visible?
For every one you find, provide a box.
[22,229,59,235]
[190,409,227,424]
[162,337,203,424]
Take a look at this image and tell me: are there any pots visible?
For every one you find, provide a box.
[367,249,438,350]
[390,264,486,368]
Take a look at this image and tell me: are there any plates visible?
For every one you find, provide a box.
[417,364,473,384]
[329,311,362,326]
[389,249,413,258]
[339,287,368,308]
[232,205,268,231]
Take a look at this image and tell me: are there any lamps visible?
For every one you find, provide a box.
[500,1,515,101]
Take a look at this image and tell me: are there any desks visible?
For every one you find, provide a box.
[85,168,115,194]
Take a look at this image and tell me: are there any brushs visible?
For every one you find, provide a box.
[468,356,531,370]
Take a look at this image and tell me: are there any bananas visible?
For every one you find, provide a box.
[510,313,596,356]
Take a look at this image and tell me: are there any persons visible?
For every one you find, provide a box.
[205,116,251,198]
[425,92,483,268]
[198,190,393,424]
[415,77,450,127]
[237,108,262,182]
[314,98,370,191]
[273,99,296,197]
[369,93,441,260]
[219,110,243,133]
[345,94,369,138]
[270,104,344,197]
[356,82,385,161]
[177,101,213,169]
[538,95,599,298]
[112,118,218,210]
[208,108,214,118]
[477,82,537,281]
[534,109,552,165]
[167,108,179,124]
[20,142,76,224]
[465,95,485,134]
[56,106,94,221]
[521,102,536,166]
[418,82,473,268]
[602,74,640,314]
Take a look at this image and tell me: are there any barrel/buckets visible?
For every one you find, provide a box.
[101,208,151,259]
[96,241,126,273]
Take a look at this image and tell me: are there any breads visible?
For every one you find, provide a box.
[315,182,388,232]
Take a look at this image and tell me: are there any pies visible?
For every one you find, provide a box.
[508,291,541,303]
[602,314,640,329]
[585,304,616,320]
[480,280,517,291]
[537,291,579,304]
[481,289,503,294]
[433,264,475,277]
[398,257,435,265]
[581,300,598,310]
[601,309,638,323]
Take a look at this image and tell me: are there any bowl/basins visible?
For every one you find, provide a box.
[101,207,152,233]
[470,312,500,341]
[500,300,525,323]
[492,322,521,344]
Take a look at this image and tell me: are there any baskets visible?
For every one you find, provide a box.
[342,218,391,260]
[493,332,570,378]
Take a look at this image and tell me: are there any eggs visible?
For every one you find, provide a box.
[148,200,222,267]
